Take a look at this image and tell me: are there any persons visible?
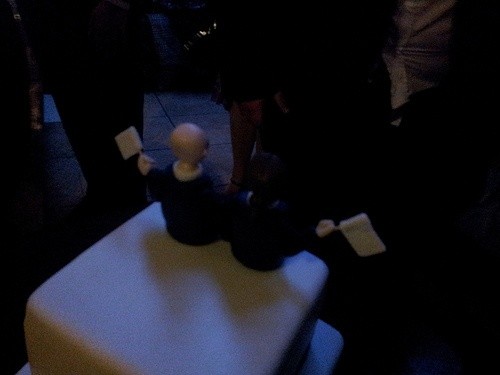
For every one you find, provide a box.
[134,122,224,246]
[228,155,338,273]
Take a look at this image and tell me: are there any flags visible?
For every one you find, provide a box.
[339,213,387,258]
[112,126,143,160]
